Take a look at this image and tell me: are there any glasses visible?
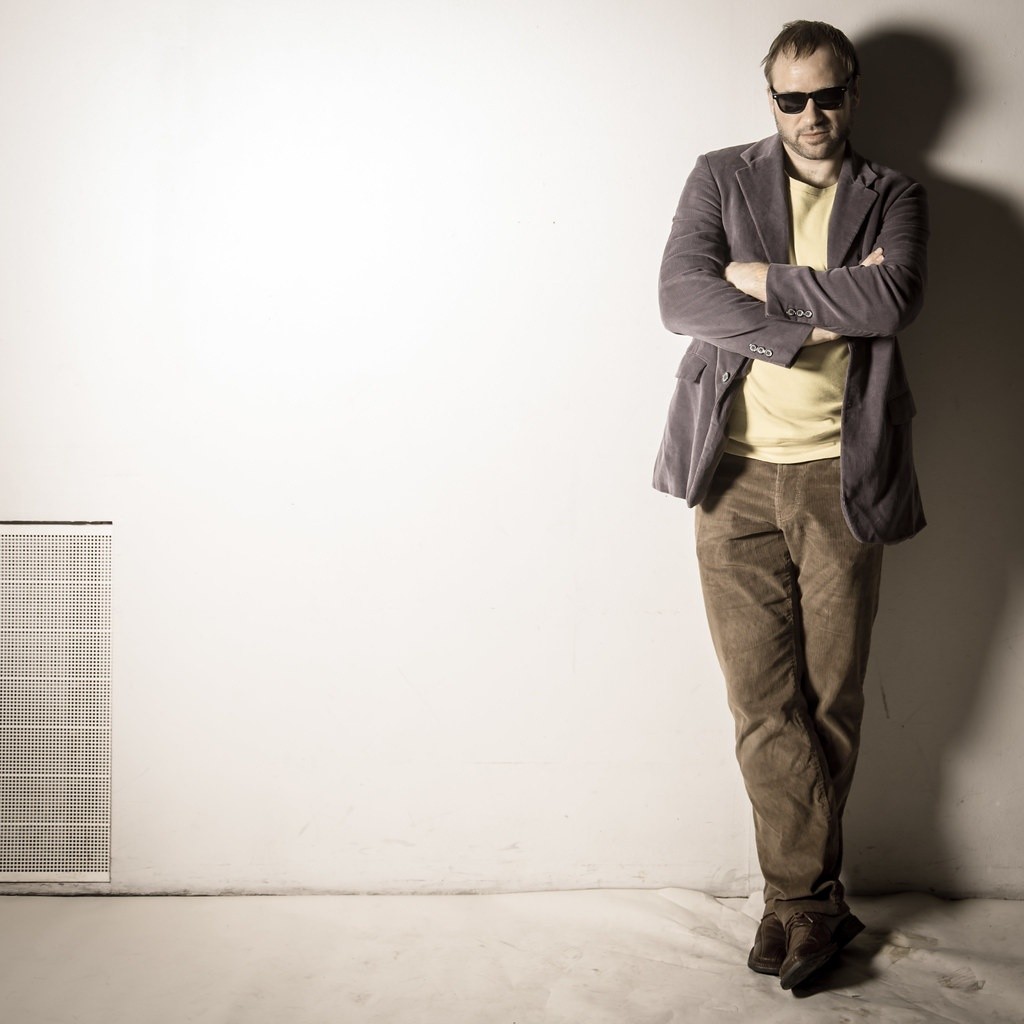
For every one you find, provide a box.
[771,81,851,115]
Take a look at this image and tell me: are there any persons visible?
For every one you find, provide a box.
[655,23,926,986]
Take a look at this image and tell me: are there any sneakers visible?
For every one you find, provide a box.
[748,912,787,975]
[779,910,865,990]
